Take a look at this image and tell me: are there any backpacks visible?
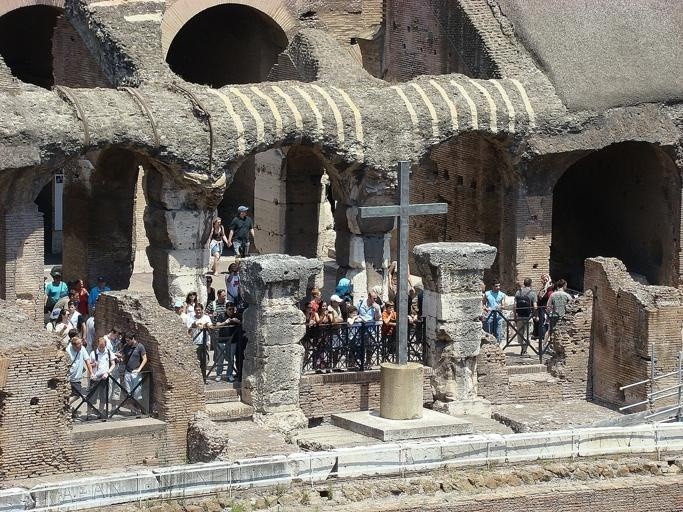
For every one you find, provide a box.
[516,289,532,317]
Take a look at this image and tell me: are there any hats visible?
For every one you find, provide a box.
[174,301,183,308]
[331,295,343,303]
[52,270,62,278]
[237,205,248,212]
[50,306,63,321]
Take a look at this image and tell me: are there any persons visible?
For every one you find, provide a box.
[174,263,248,381]
[531,274,555,340]
[512,277,540,358]
[228,206,254,258]
[545,278,573,330]
[302,277,424,370]
[207,216,230,276]
[482,279,509,344]
[44,272,148,420]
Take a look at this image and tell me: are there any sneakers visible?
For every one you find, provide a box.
[216,373,238,382]
[310,364,375,375]
[519,343,555,357]
[75,406,141,424]
